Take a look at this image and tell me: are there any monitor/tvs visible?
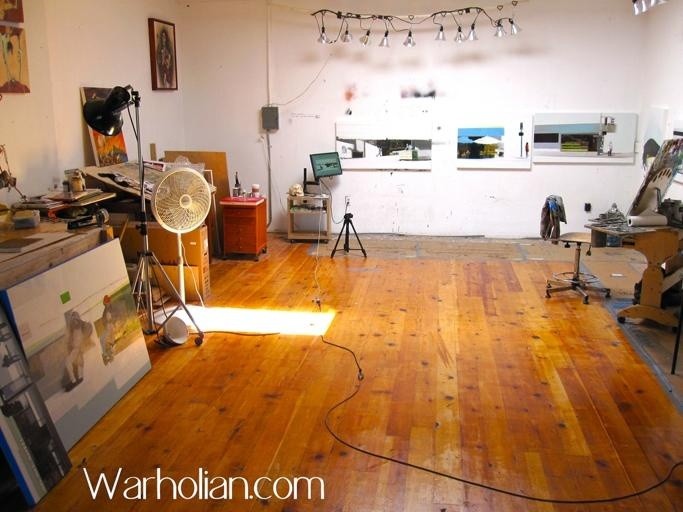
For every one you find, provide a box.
[310,152,343,180]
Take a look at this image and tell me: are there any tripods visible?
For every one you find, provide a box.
[331,214,369,258]
[128,216,205,350]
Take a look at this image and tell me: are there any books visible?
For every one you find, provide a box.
[10,184,117,213]
[219,195,265,205]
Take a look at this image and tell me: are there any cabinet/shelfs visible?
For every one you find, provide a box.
[286,193,331,244]
[219,195,268,262]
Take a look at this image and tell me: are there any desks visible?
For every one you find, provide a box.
[1,222,114,290]
[584,225,682,328]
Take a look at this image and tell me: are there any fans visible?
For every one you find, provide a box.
[152,167,213,325]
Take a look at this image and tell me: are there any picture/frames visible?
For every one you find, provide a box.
[148,17,178,91]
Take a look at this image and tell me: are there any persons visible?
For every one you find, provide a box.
[61,311,93,392]
[156,30,175,88]
[99,295,116,366]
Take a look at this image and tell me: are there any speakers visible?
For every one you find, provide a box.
[262,107,279,132]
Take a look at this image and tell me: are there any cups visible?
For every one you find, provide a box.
[251,184,260,198]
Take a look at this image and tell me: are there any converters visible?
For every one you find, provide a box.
[584,202,591,211]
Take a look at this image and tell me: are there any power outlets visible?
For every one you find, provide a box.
[345,196,352,206]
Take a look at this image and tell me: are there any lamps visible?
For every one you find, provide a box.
[629,0,667,15]
[310,0,521,47]
[83,86,131,136]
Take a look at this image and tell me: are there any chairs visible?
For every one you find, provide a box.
[545,197,610,304]
